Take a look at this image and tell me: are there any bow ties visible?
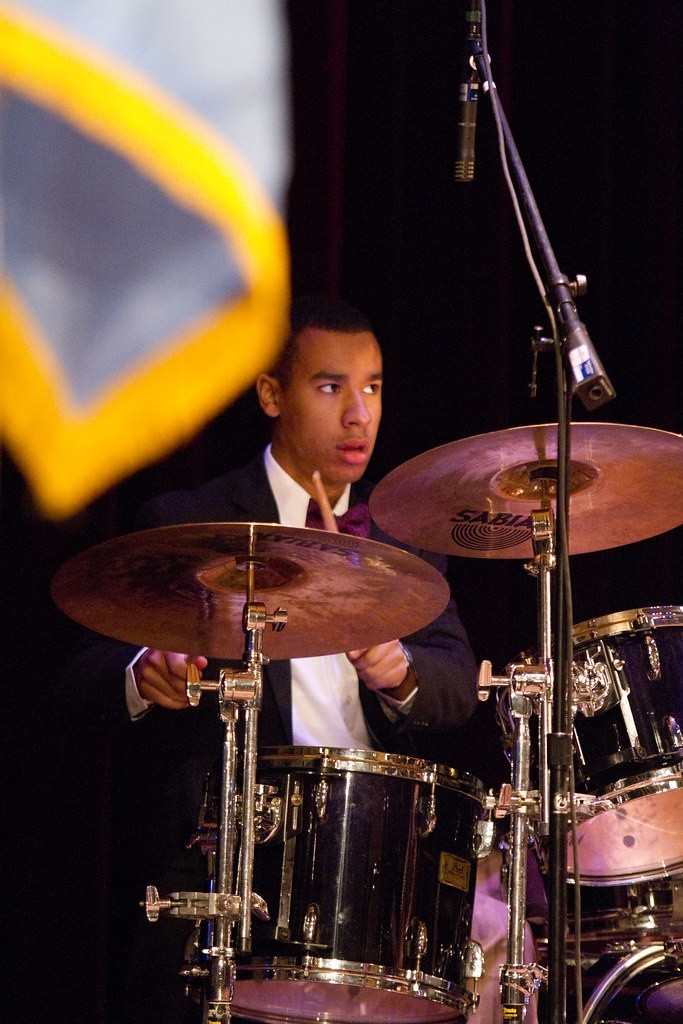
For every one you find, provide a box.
[305,498,370,539]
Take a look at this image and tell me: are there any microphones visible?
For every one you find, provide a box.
[455,0,482,181]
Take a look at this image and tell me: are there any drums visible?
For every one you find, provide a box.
[569,603,683,890]
[570,935,683,1024]
[174,742,495,1024]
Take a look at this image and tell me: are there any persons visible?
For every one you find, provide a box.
[59,295,549,1024]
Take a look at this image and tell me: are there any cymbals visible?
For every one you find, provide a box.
[367,417,683,561]
[46,519,453,663]
